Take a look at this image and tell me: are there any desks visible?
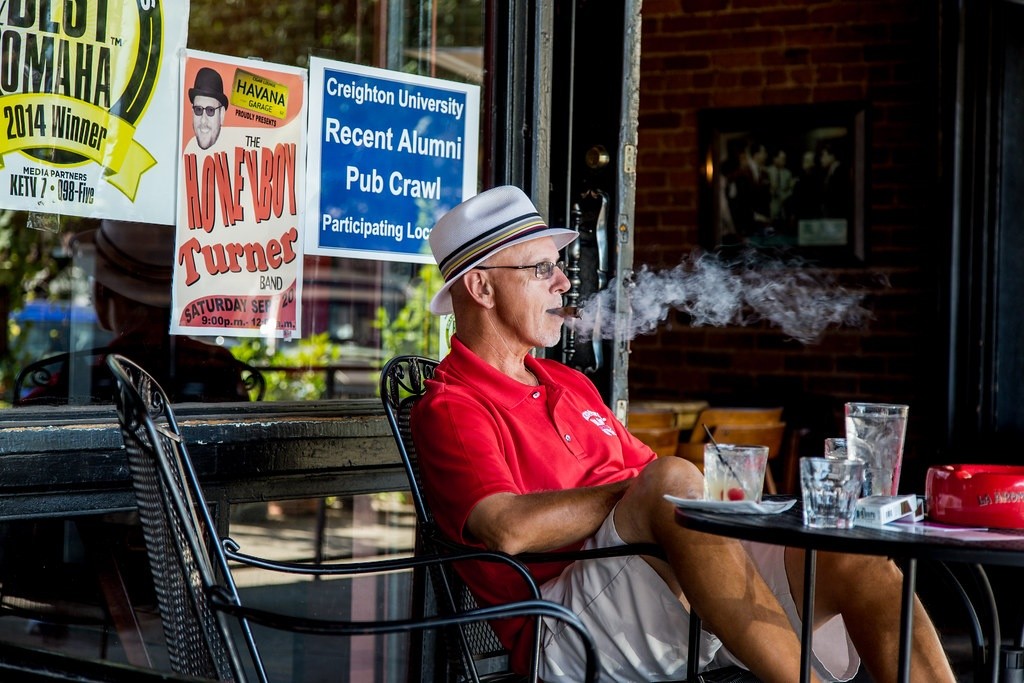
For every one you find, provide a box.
[673,491,1024,683]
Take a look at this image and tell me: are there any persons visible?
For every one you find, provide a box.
[718,138,846,243]
[187,67,228,151]
[19,222,250,402]
[407,183,955,683]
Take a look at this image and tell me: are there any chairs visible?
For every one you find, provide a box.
[623,409,680,460]
[380,354,753,683]
[678,404,788,495]
[105,350,603,683]
[10,340,268,606]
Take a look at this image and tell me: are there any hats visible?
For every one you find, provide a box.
[70,221,175,310]
[188,68,228,110]
[431,185,580,315]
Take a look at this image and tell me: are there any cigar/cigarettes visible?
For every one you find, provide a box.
[546,307,584,319]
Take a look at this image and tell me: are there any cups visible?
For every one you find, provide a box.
[846,403,908,497]
[704,443,769,506]
[799,458,864,531]
[824,438,872,485]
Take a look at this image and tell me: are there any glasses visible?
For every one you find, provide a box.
[473,261,564,280]
[193,106,223,116]
